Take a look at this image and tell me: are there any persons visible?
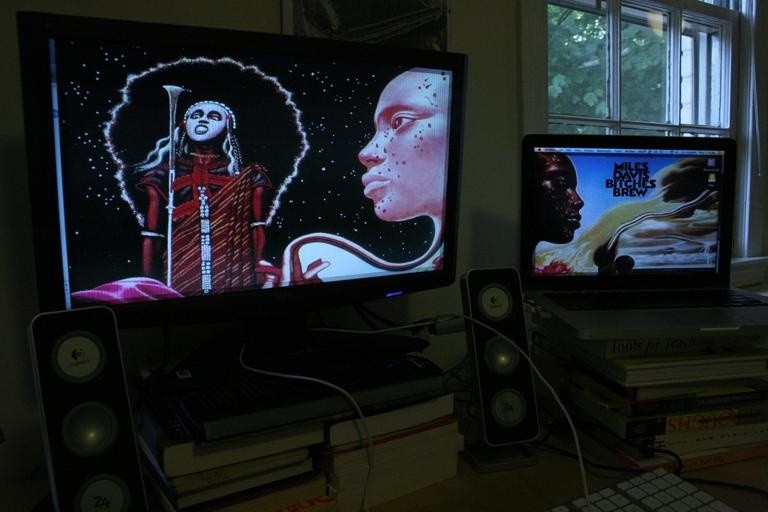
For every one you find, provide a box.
[532,153,584,270]
[282,66,453,287]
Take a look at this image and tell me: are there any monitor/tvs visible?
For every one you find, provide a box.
[16,10,469,391]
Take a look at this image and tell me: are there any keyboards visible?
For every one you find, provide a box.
[544,467,739,512]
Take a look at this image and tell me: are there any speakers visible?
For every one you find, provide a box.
[27,305,150,512]
[459,265,542,475]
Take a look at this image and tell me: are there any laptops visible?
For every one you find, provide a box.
[520,134,768,341]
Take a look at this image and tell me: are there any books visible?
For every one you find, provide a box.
[529,310,768,475]
[134,391,464,512]
